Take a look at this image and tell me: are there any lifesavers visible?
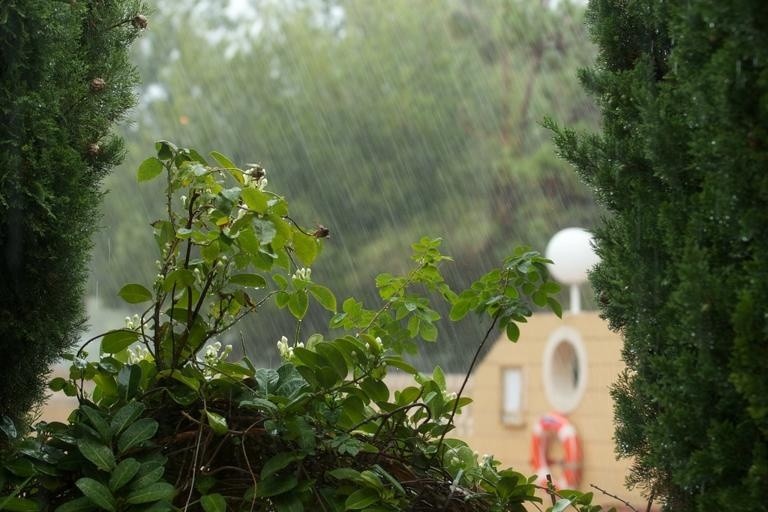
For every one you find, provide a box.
[529,415,582,496]
